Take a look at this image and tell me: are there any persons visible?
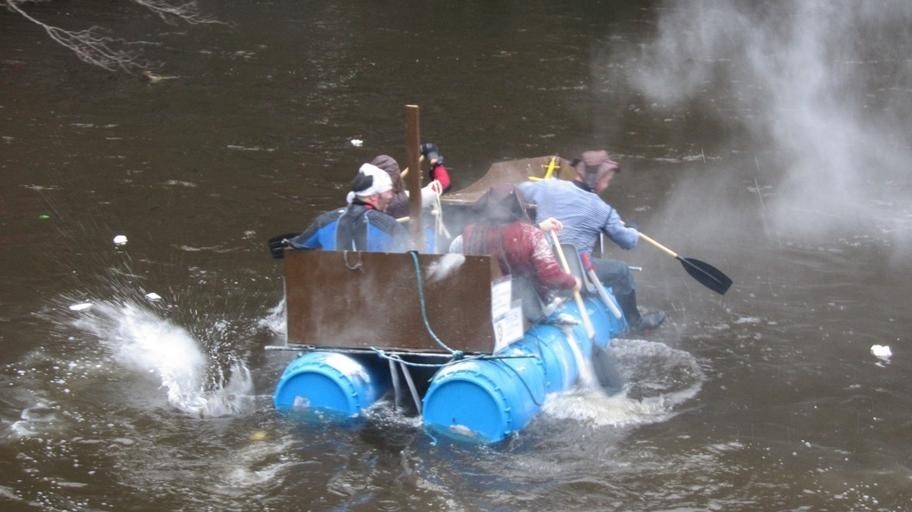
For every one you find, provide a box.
[450,183,581,324]
[514,148,668,336]
[368,142,451,252]
[288,162,416,252]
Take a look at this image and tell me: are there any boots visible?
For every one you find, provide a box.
[615,289,667,333]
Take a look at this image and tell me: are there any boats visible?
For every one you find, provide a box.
[264,266,642,448]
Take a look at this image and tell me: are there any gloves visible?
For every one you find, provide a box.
[420,142,441,162]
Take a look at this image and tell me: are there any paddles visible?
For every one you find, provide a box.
[631,230,733,296]
[548,229,622,397]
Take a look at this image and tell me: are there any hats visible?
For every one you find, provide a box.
[370,154,406,193]
[485,186,517,224]
[575,149,621,189]
